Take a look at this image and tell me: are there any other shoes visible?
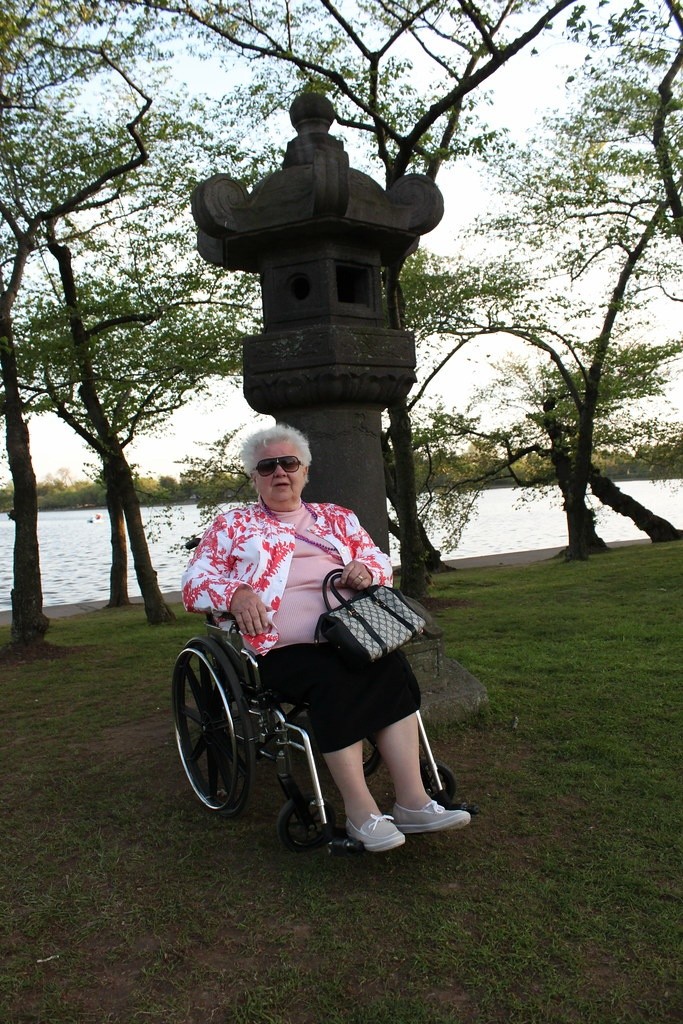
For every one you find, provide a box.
[345,813,406,852]
[393,800,471,833]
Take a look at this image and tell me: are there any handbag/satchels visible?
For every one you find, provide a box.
[315,568,427,671]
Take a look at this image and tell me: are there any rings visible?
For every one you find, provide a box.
[357,575,364,581]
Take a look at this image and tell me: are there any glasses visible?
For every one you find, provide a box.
[253,456,303,477]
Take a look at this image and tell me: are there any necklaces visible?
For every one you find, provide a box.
[262,499,342,561]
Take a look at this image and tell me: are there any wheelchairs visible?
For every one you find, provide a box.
[171,537,481,853]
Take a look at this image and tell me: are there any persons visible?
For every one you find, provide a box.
[181,426,471,852]
